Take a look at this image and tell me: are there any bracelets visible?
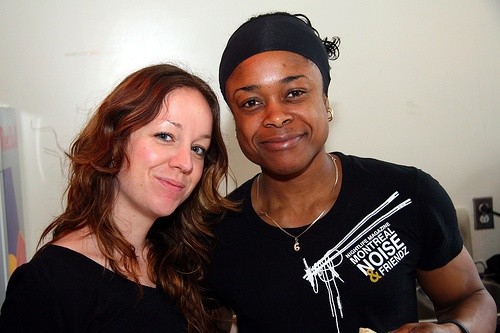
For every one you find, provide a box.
[435,319,470,333]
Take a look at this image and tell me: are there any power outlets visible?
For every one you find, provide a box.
[472,196,495,231]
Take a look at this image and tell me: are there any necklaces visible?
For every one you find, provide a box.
[256,152,339,252]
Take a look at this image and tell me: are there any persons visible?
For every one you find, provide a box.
[204,11,498,333]
[0,60,247,333]
